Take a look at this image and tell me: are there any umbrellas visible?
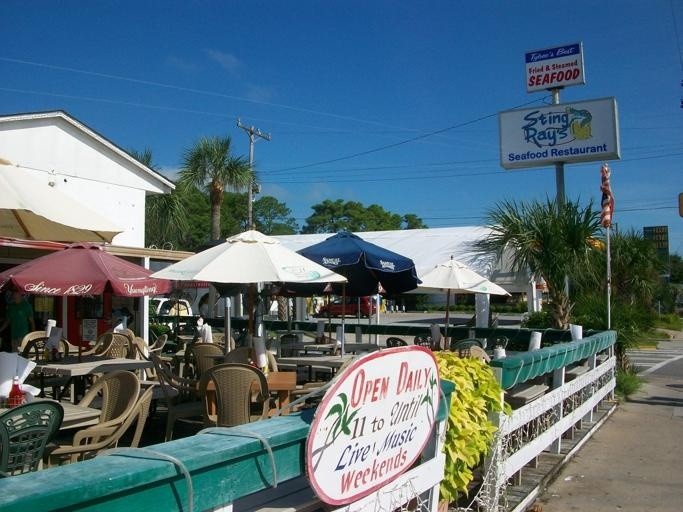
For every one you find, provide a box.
[147,228,349,363]
[0,156,124,245]
[0,238,173,365]
[405,254,513,350]
[281,226,422,358]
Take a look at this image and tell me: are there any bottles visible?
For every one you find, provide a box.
[52,344,59,360]
[8,377,23,409]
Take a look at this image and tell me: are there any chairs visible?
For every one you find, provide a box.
[0,329,510,479]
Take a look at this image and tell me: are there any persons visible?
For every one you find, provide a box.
[269,294,279,320]
[190,312,213,344]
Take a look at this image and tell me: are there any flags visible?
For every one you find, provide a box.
[599,162,615,231]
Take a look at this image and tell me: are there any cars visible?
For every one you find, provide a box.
[148,296,195,333]
[320,294,380,320]
